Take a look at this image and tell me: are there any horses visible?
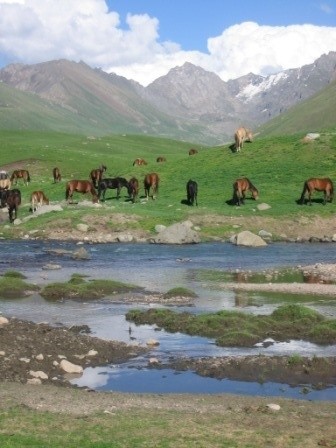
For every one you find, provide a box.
[189,148,198,155]
[298,178,334,205]
[233,178,259,206]
[0,168,62,222]
[234,127,253,152]
[156,157,165,162]
[65,159,159,204]
[185,179,198,206]
